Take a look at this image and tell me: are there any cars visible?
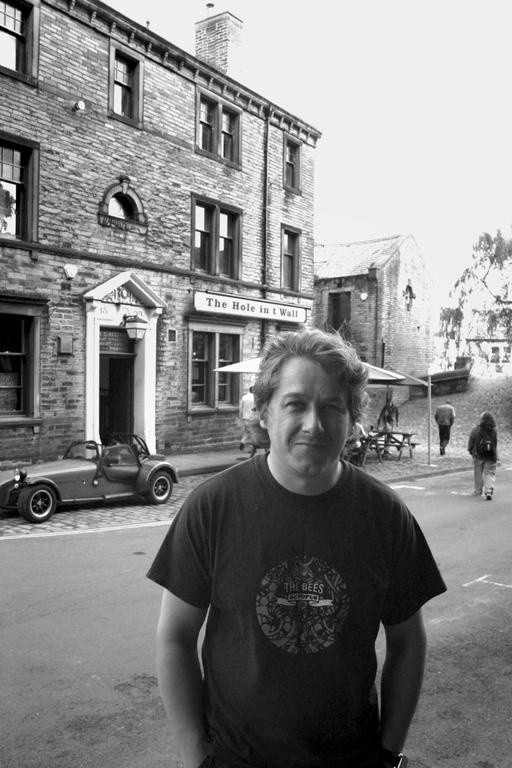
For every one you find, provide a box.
[0,433,181,523]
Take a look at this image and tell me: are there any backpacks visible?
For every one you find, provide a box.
[476,424,496,457]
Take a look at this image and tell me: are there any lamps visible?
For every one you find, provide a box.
[69,100,86,112]
[124,310,148,340]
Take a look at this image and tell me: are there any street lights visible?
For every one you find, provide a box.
[423,349,436,466]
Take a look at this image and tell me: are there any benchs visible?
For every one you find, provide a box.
[390,441,421,458]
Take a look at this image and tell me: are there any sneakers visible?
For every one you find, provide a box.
[473,492,492,499]
[440,447,445,455]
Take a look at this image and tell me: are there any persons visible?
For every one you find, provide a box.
[376,394,399,454]
[238,384,258,457]
[434,397,458,455]
[144,324,447,767]
[346,414,371,464]
[468,410,498,498]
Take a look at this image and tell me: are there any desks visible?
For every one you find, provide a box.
[361,428,416,464]
